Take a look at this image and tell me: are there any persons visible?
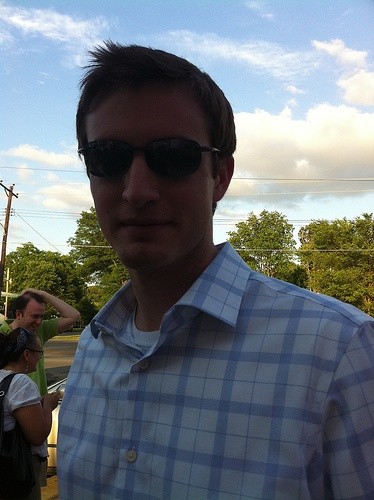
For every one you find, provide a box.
[0,328,60,500]
[0,288,82,487]
[56,40,374,500]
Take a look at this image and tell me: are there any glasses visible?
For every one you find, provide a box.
[77,135,221,180]
[24,347,44,358]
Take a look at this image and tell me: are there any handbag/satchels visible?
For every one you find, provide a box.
[0,372,36,500]
